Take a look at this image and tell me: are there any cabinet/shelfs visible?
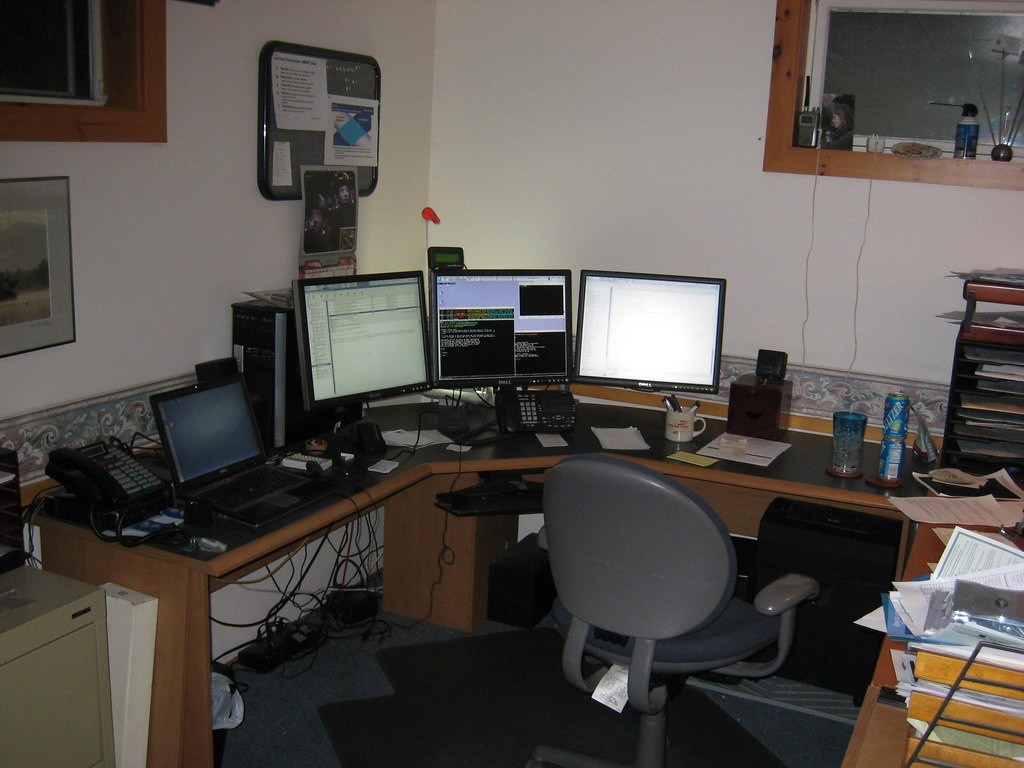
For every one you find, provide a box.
[940,278,1024,494]
[0,565,115,768]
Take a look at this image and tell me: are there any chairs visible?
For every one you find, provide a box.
[541,456,822,768]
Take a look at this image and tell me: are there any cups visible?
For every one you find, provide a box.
[831,411,868,473]
[868,138,885,153]
[665,406,707,443]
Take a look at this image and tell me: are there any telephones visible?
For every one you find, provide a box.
[498,386,576,433]
[46,437,170,514]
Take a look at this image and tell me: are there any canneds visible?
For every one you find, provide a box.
[878,391,909,478]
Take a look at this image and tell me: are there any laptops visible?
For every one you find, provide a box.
[149,372,338,530]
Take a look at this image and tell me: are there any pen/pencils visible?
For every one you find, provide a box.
[662,394,700,414]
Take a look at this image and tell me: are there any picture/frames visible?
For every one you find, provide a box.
[0,176,76,359]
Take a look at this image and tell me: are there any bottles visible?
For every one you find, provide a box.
[953,112,979,159]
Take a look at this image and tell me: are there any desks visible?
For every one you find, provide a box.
[21,392,1023,768]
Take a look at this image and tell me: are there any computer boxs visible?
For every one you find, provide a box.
[231,298,362,447]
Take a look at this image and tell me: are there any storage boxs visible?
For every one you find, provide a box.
[98,579,160,767]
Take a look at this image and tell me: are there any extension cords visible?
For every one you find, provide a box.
[238,619,307,666]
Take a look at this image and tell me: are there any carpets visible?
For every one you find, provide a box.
[320,626,782,768]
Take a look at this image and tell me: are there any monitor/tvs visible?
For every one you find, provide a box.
[572,270,727,395]
[291,270,433,412]
[429,268,574,389]
